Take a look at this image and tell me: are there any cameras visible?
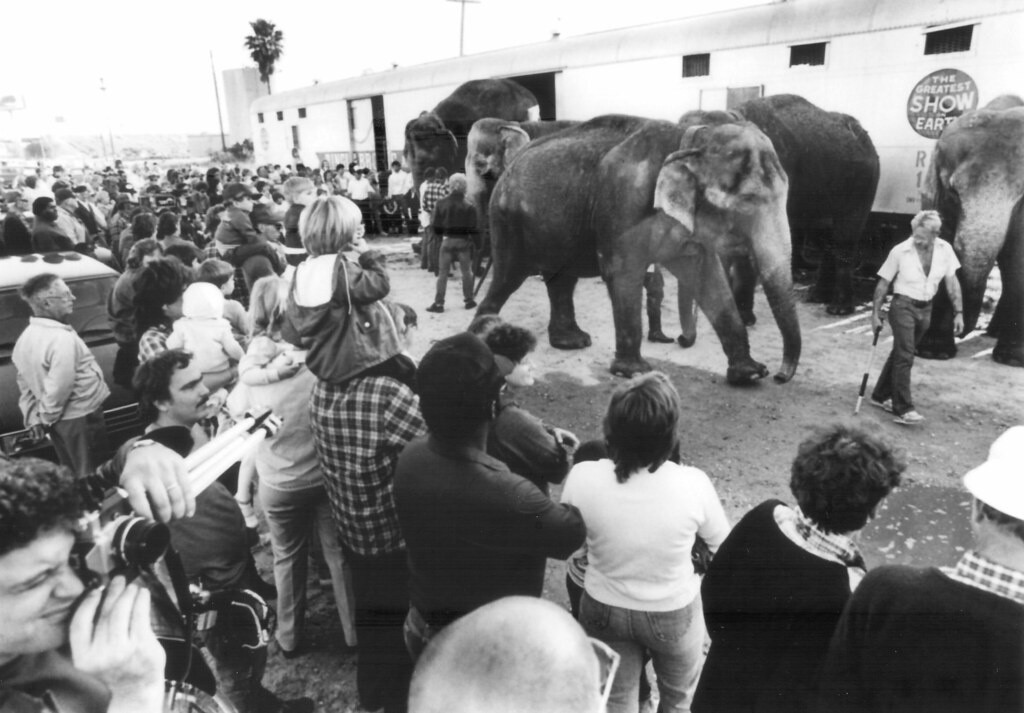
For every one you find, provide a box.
[68,487,169,588]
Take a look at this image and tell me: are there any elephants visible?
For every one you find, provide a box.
[404,77,540,194]
[915,95,1024,367]
[462,114,589,277]
[677,93,880,315]
[467,114,803,385]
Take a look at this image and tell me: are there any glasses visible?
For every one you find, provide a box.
[44,292,74,303]
[586,637,619,713]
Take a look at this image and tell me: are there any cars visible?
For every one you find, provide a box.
[1,249,153,477]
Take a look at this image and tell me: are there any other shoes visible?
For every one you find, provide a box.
[466,301,477,309]
[275,636,295,659]
[276,697,316,713]
[317,576,330,588]
[426,302,444,313]
[235,497,259,529]
[347,641,358,650]
[252,581,279,601]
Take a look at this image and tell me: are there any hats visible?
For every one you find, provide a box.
[961,426,1023,521]
[223,184,263,208]
[352,165,362,174]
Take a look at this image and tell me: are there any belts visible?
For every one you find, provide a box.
[894,294,931,308]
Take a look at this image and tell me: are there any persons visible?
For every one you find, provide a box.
[865,207,966,430]
[0,160,1024,713]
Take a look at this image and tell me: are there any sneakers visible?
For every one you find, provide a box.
[893,411,926,425]
[871,398,893,412]
